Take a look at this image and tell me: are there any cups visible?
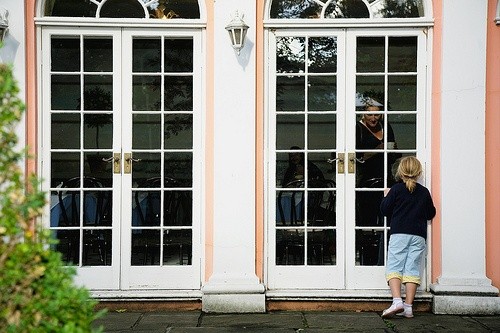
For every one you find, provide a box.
[387,142,396,148]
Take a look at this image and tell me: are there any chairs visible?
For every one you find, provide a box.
[276,176,383,267]
[135,177,191,266]
[58,177,112,264]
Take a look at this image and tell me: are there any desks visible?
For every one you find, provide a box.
[276,191,305,224]
[49,192,147,249]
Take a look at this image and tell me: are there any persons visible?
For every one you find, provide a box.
[356,88,403,266]
[380,156,437,317]
[283,146,326,221]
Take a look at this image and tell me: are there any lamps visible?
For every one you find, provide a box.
[225,9,250,56]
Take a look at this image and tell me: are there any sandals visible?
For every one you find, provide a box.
[381,298,405,318]
[395,303,414,317]
